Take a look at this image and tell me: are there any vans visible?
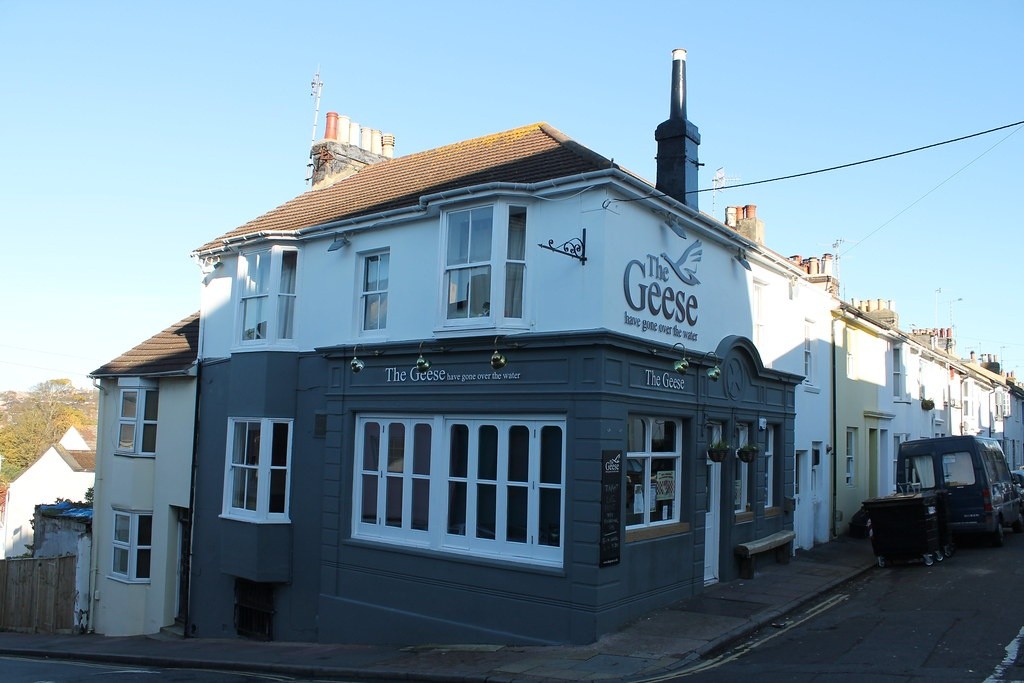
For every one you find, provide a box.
[896,435,1024,549]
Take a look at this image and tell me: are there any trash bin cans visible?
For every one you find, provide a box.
[860,491,953,568]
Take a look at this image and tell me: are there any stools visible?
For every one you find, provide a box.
[735,529,799,580]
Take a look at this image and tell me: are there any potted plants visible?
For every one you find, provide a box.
[704,422,731,462]
[734,424,760,464]
[920,396,935,411]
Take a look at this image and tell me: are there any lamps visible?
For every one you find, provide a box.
[687,350,722,382]
[415,340,455,373]
[490,335,529,370]
[649,342,690,375]
[350,343,386,374]
